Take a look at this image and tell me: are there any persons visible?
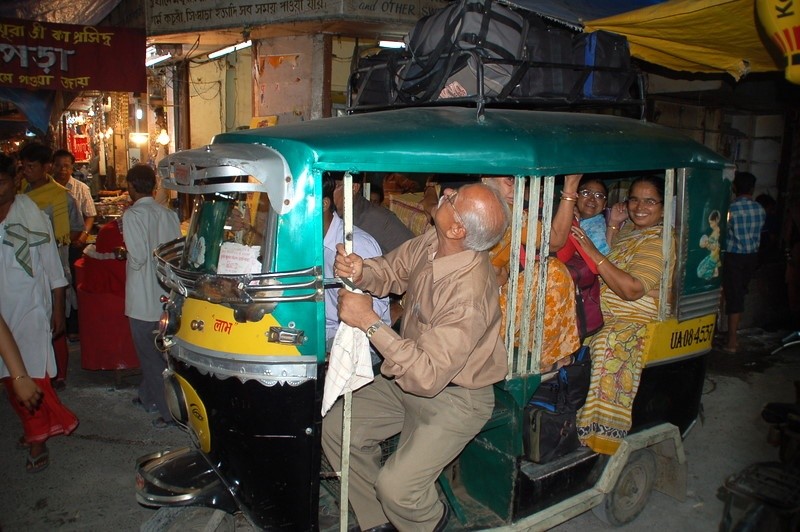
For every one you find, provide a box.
[710,171,768,351]
[0,139,98,469]
[112,161,187,431]
[191,168,678,532]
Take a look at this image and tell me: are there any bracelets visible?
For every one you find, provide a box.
[595,256,608,268]
[606,225,621,231]
[12,370,27,382]
[560,196,576,204]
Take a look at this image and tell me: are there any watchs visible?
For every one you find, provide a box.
[365,319,384,337]
[84,231,89,236]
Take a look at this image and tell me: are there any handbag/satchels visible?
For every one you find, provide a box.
[523,407,580,463]
[356,0,631,106]
[528,346,591,413]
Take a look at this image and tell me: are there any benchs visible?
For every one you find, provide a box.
[392,351,587,458]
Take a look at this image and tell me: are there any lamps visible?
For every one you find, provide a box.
[145,42,183,66]
[207,39,251,60]
[378,38,405,49]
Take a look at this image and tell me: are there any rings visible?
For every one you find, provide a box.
[580,236,584,239]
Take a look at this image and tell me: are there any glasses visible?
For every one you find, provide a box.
[628,198,661,206]
[578,190,606,201]
[446,190,464,223]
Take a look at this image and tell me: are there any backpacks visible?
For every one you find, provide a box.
[519,218,604,339]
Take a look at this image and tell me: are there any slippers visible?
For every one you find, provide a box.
[25,448,50,472]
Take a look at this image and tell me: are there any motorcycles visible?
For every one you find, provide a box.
[135,47,736,532]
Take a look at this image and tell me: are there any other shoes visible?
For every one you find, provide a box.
[726,342,741,355]
